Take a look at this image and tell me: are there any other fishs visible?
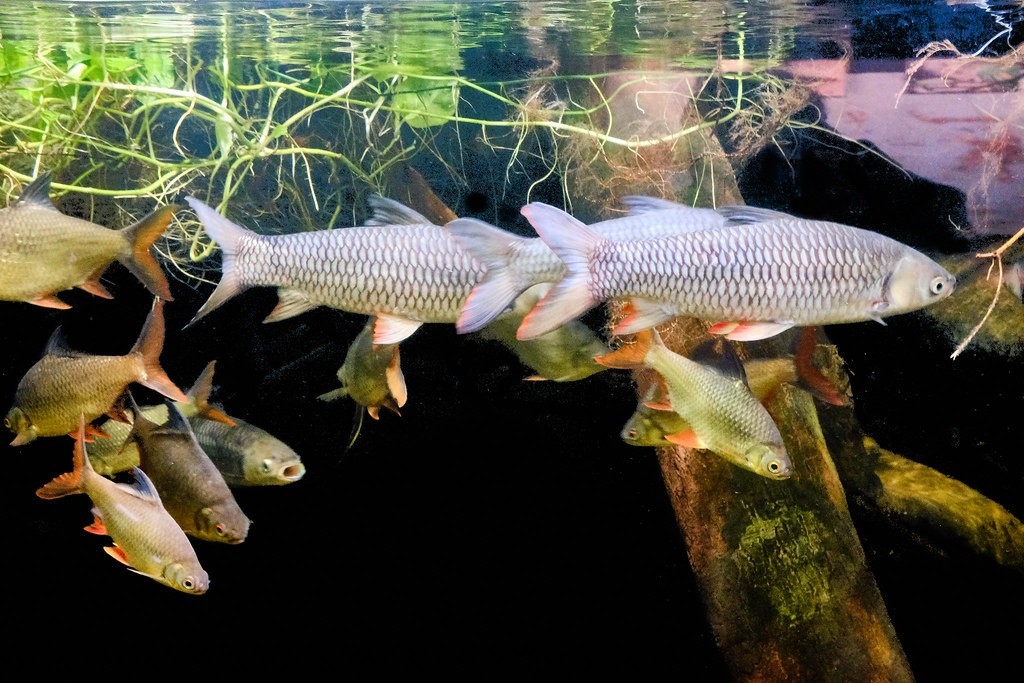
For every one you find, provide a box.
[3,293,307,597]
[182,193,960,482]
[0,169,175,310]
